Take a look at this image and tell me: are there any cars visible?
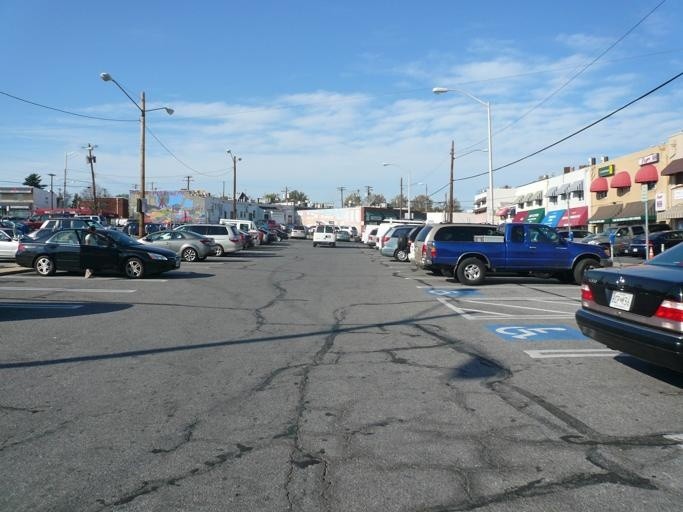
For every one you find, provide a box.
[558,230,592,243]
[135,229,215,261]
[575,242,682,380]
[0,207,167,262]
[628,230,682,258]
[15,228,180,277]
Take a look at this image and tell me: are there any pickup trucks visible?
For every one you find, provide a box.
[424,223,613,286]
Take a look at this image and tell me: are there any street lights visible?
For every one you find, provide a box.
[47,173,55,209]
[383,162,410,219]
[432,85,494,226]
[226,148,241,220]
[99,72,174,239]
[418,182,427,219]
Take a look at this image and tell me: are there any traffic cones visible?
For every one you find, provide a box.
[647,246,653,260]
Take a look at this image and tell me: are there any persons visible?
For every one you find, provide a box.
[83,225,100,278]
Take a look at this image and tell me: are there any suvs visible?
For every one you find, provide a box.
[290,223,358,247]
[360,219,500,277]
[168,218,294,258]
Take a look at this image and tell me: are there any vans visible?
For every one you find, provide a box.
[581,223,670,259]
